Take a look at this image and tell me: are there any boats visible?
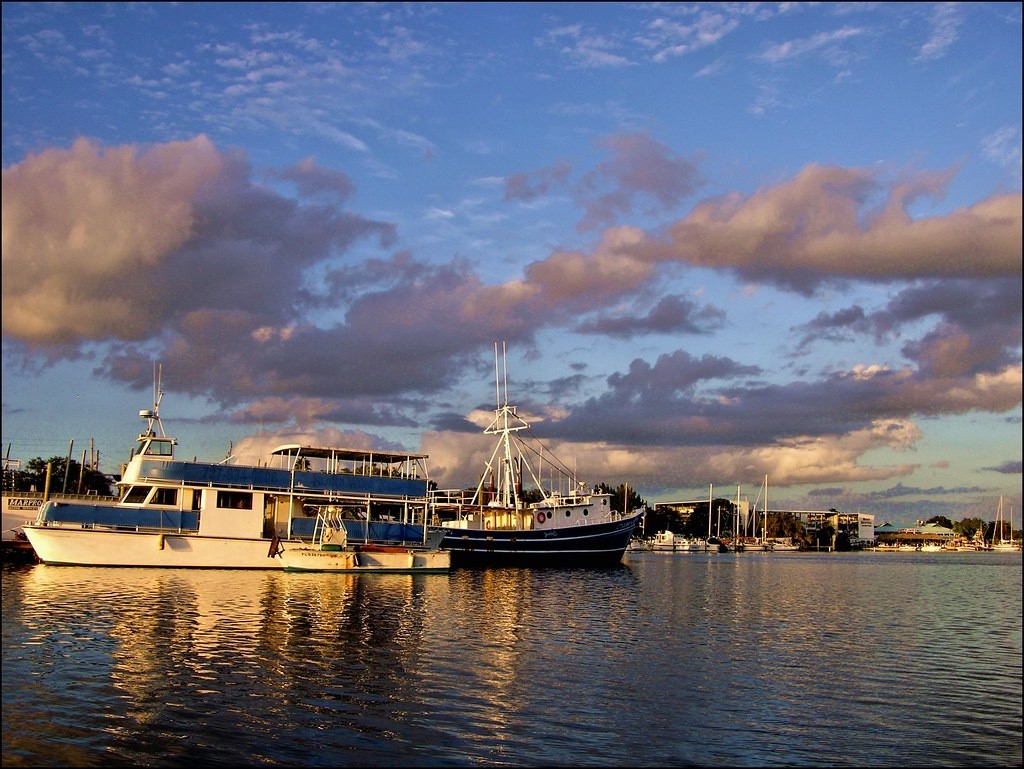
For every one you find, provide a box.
[866,543,976,551]
[442,341,647,567]
[651,531,721,553]
[23,360,453,573]
[731,543,801,550]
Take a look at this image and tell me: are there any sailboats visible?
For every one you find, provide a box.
[983,493,1020,551]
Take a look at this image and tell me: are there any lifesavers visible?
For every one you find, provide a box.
[537,512,546,523]
[158,534,164,550]
[486,520,490,529]
[321,544,342,551]
